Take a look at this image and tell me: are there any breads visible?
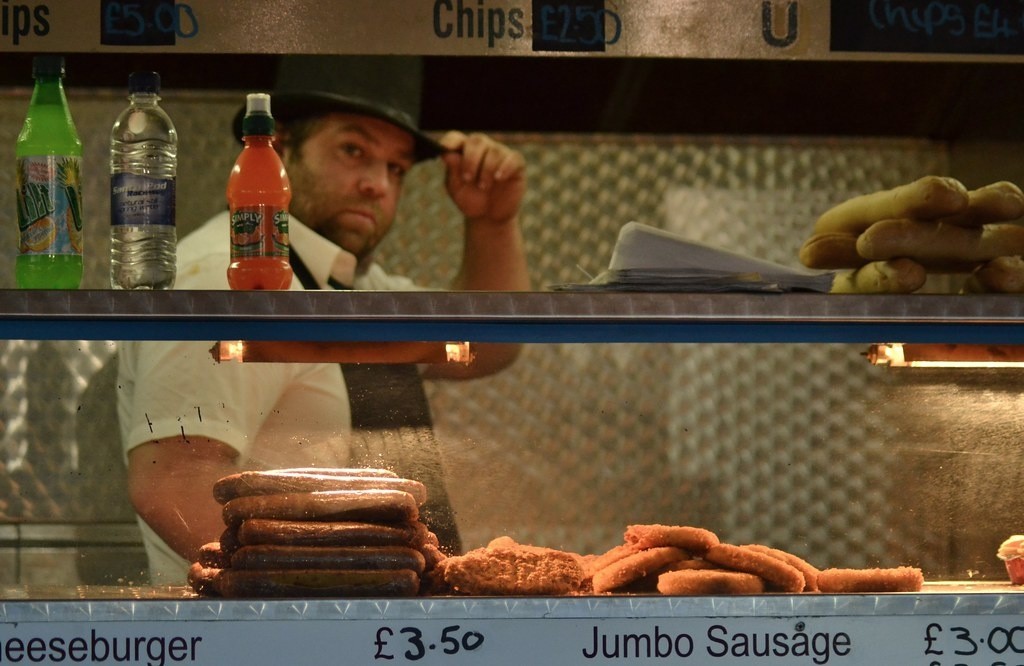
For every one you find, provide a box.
[796,176,1024,296]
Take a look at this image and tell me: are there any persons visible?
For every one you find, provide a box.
[117,91,533,585]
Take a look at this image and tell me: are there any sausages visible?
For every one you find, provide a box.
[189,466,446,596]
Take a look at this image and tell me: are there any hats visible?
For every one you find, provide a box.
[230,54,445,164]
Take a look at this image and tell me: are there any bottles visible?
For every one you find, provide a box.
[13,54,83,289]
[110,72,177,290]
[227,93,293,290]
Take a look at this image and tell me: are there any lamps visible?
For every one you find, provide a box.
[860,342,1023,369]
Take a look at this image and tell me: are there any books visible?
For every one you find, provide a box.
[543,221,836,293]
